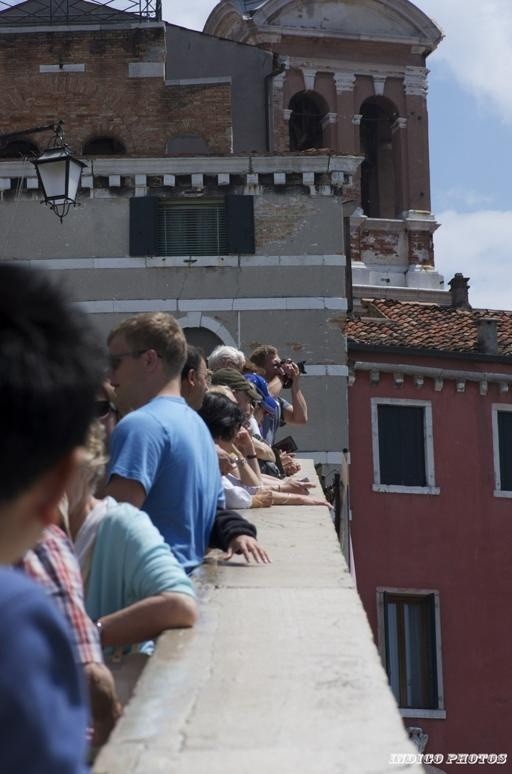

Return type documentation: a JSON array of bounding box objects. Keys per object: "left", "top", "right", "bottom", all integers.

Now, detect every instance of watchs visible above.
[
  {"left": 247, "top": 455, "right": 256, "bottom": 459},
  {"left": 235, "top": 458, "right": 247, "bottom": 468}
]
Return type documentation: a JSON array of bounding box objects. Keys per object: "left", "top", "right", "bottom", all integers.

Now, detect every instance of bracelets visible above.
[
  {"left": 277, "top": 484, "right": 281, "bottom": 494},
  {"left": 283, "top": 491, "right": 291, "bottom": 506}
]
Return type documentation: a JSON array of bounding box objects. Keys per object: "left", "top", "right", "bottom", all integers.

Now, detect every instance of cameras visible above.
[{"left": 272, "top": 359, "right": 307, "bottom": 381}]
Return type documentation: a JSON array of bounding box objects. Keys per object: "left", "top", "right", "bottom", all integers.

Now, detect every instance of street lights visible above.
[{"left": 0, "top": 119, "right": 88, "bottom": 224}]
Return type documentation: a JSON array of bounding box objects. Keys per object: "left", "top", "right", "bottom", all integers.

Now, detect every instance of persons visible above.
[
  {"left": 64, "top": 420, "right": 196, "bottom": 661},
  {"left": 102, "top": 313, "right": 220, "bottom": 576},
  {"left": 16, "top": 446, "right": 122, "bottom": 747},
  {"left": 173, "top": 338, "right": 333, "bottom": 565},
  {"left": 0, "top": 253, "right": 97, "bottom": 769}
]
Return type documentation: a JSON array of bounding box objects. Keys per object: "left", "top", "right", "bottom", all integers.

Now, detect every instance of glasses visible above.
[{"left": 106, "top": 352, "right": 146, "bottom": 369}]
